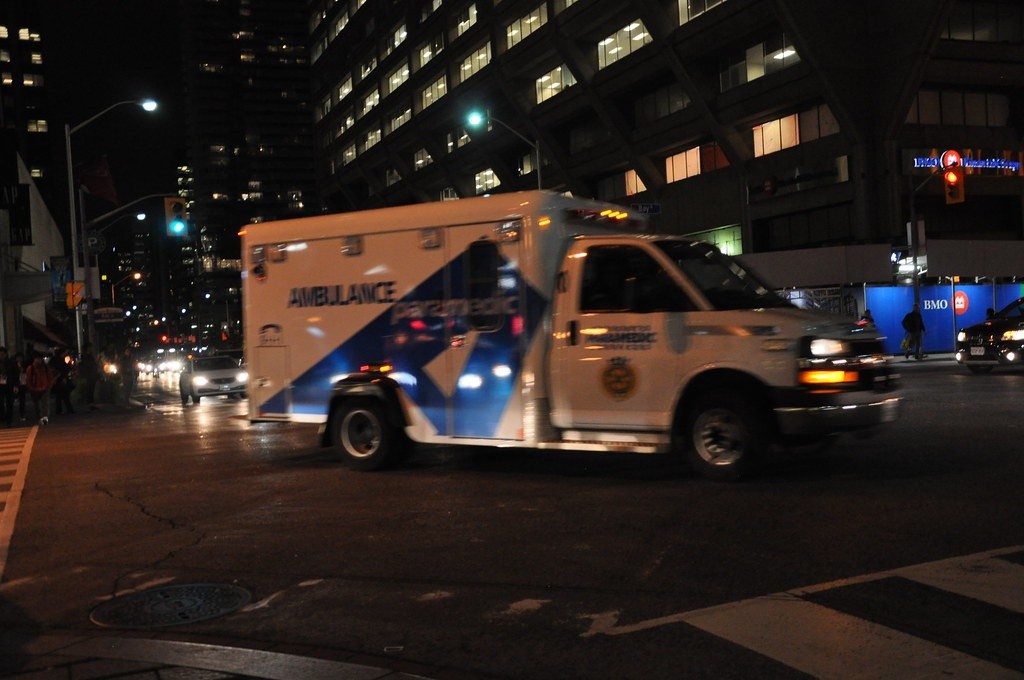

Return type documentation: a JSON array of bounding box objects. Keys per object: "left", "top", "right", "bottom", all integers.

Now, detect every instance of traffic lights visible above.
[{"left": 945, "top": 169, "right": 964, "bottom": 204}]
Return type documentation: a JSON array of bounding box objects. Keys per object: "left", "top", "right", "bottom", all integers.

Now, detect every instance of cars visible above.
[
  {"left": 180, "top": 355, "right": 247, "bottom": 405},
  {"left": 955, "top": 297, "right": 1024, "bottom": 373}
]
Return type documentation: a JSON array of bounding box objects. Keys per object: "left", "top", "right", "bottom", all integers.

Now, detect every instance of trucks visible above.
[{"left": 239, "top": 189, "right": 904, "bottom": 481}]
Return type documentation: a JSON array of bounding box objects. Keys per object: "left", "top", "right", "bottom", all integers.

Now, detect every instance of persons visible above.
[
  {"left": 592, "top": 255, "right": 646, "bottom": 308},
  {"left": 861, "top": 310, "right": 875, "bottom": 327},
  {"left": 0, "top": 343, "right": 76, "bottom": 426},
  {"left": 902, "top": 304, "right": 927, "bottom": 359},
  {"left": 81, "top": 343, "right": 100, "bottom": 412},
  {"left": 121, "top": 347, "right": 138, "bottom": 410}
]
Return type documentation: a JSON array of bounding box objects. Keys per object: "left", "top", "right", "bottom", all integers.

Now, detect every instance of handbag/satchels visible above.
[{"left": 900, "top": 334, "right": 911, "bottom": 351}]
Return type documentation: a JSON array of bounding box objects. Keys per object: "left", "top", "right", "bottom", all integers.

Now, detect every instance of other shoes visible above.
[
  {"left": 905, "top": 353, "right": 908, "bottom": 358},
  {"left": 915, "top": 355, "right": 919, "bottom": 359}
]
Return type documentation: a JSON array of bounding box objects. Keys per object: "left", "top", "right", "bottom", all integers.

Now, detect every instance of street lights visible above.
[
  {"left": 64, "top": 100, "right": 157, "bottom": 358},
  {"left": 165, "top": 198, "right": 187, "bottom": 237},
  {"left": 469, "top": 113, "right": 542, "bottom": 190},
  {"left": 111, "top": 273, "right": 141, "bottom": 305}
]
